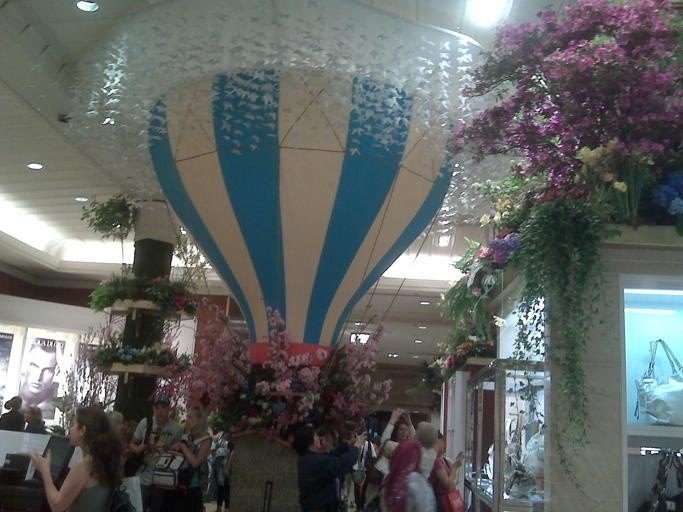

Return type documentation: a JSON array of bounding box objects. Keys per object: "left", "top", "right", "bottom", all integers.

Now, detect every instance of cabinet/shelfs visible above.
[{"left": 461, "top": 358, "right": 545, "bottom": 511}]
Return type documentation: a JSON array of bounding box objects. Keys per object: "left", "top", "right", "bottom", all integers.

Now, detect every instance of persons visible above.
[
  {"left": 17, "top": 337, "right": 77, "bottom": 420},
  {"left": 278, "top": 406, "right": 463, "bottom": 512},
  {"left": 22, "top": 406, "right": 47, "bottom": 434},
  {"left": 0, "top": 396, "right": 24, "bottom": 431},
  {"left": 28, "top": 390, "right": 235, "bottom": 510}
]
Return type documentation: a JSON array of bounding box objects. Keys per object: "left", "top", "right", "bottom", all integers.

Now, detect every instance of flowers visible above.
[
  {"left": 78, "top": 190, "right": 137, "bottom": 242},
  {"left": 86, "top": 276, "right": 198, "bottom": 375},
  {"left": 165, "top": 296, "right": 393, "bottom": 459}
]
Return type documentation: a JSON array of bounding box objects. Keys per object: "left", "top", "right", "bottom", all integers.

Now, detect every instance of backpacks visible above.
[{"left": 152, "top": 433, "right": 212, "bottom": 491}]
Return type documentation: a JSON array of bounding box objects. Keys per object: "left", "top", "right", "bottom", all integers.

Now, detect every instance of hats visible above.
[{"left": 154, "top": 394, "right": 171, "bottom": 405}]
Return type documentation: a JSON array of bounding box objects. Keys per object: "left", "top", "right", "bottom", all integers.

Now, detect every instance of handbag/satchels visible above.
[
  {"left": 124, "top": 449, "right": 144, "bottom": 477},
  {"left": 635, "top": 340, "right": 683, "bottom": 426},
  {"left": 365, "top": 457, "right": 384, "bottom": 486},
  {"left": 440, "top": 490, "right": 466, "bottom": 512},
  {"left": 637, "top": 450, "right": 682, "bottom": 512},
  {"left": 105, "top": 478, "right": 137, "bottom": 512}
]
